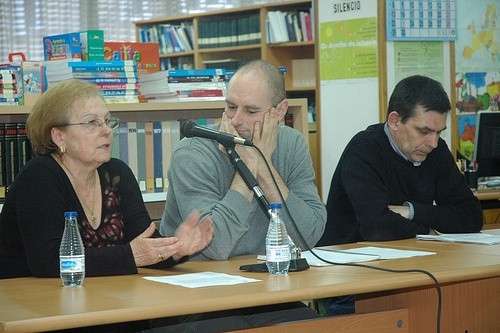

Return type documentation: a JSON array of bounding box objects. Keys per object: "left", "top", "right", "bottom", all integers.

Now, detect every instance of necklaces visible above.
[{"left": 57, "top": 154, "right": 97, "bottom": 225}]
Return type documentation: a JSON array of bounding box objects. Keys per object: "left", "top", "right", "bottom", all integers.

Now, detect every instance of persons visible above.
[
  {"left": 0, "top": 81, "right": 214, "bottom": 279},
  {"left": 159, "top": 59, "right": 329, "bottom": 333},
  {"left": 314, "top": 76, "right": 484, "bottom": 315}
]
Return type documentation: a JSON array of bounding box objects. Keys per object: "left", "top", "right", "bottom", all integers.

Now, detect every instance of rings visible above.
[{"left": 160, "top": 253, "right": 165, "bottom": 258}]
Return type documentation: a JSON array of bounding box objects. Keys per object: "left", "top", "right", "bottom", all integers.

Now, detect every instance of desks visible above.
[{"left": 0, "top": 227, "right": 500, "bottom": 333}]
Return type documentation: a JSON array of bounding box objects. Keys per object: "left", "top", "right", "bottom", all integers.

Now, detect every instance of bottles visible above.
[
  {"left": 59, "top": 211, "right": 85, "bottom": 287},
  {"left": 265, "top": 203, "right": 291, "bottom": 275}
]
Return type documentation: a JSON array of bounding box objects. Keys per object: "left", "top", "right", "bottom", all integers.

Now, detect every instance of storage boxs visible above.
[{"left": 42, "top": 30, "right": 161, "bottom": 72}]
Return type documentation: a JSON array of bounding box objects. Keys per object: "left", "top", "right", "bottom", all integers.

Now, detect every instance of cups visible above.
[{"left": 465, "top": 170, "right": 478, "bottom": 193}]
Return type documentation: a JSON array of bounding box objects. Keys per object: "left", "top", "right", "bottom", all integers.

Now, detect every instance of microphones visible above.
[{"left": 179, "top": 121, "right": 253, "bottom": 146}]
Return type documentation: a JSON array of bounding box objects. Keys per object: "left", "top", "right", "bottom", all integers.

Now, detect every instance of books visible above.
[{"left": 0, "top": 8, "right": 315, "bottom": 206}]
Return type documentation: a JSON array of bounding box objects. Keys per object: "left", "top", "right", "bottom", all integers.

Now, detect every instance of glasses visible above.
[{"left": 61, "top": 117, "right": 121, "bottom": 131}]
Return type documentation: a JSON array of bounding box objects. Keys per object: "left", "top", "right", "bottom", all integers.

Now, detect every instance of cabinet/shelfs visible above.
[{"left": 135, "top": 0, "right": 321, "bottom": 199}]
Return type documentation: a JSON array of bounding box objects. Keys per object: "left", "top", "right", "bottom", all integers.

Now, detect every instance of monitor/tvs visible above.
[{"left": 474, "top": 110, "right": 500, "bottom": 180}]
